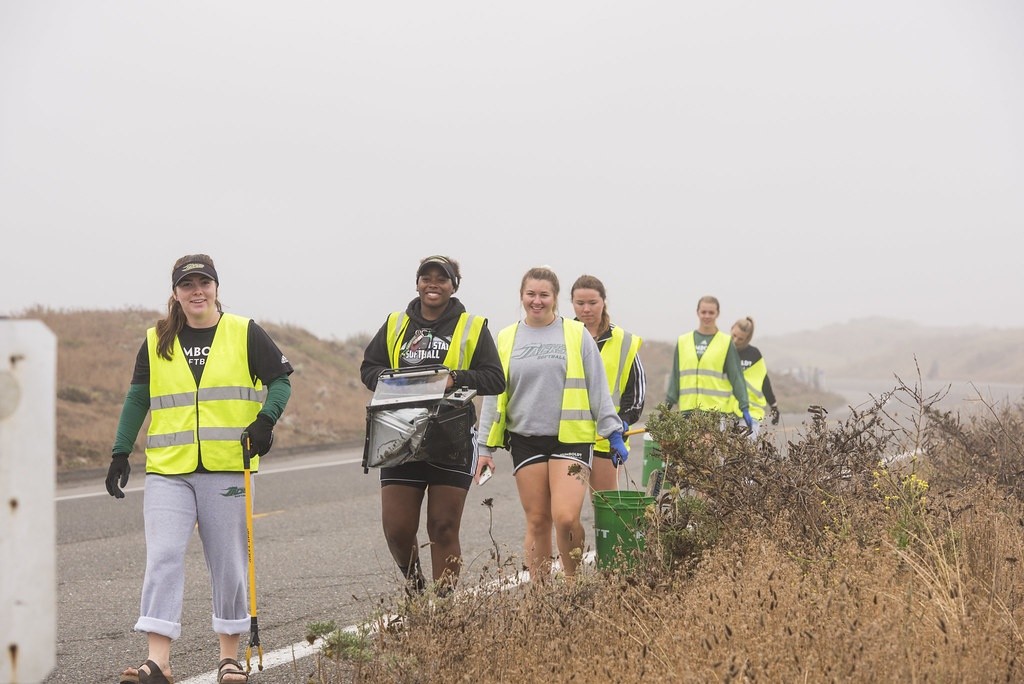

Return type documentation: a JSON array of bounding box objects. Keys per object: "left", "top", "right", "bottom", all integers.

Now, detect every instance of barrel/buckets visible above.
[
  {"left": 642, "top": 432, "right": 673, "bottom": 489},
  {"left": 592, "top": 457, "right": 655, "bottom": 571}
]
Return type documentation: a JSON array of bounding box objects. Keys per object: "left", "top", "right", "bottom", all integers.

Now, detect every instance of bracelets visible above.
[{"left": 449, "top": 372, "right": 457, "bottom": 387}]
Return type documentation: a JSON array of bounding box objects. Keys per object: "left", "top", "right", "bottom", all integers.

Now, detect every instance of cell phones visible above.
[{"left": 479, "top": 464, "right": 492, "bottom": 486}]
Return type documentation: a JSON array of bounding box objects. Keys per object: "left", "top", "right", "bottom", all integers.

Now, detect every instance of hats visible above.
[
  {"left": 415, "top": 256, "right": 457, "bottom": 289},
  {"left": 171, "top": 262, "right": 218, "bottom": 288}
]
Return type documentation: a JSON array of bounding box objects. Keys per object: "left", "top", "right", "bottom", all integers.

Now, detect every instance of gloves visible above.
[
  {"left": 742, "top": 408, "right": 753, "bottom": 427},
  {"left": 622, "top": 420, "right": 631, "bottom": 442},
  {"left": 241, "top": 413, "right": 275, "bottom": 459},
  {"left": 609, "top": 431, "right": 628, "bottom": 468},
  {"left": 105, "top": 452, "right": 131, "bottom": 498},
  {"left": 772, "top": 408, "right": 780, "bottom": 426}
]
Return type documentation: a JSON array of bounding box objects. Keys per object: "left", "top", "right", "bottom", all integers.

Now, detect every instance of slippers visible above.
[
  {"left": 217, "top": 658, "right": 248, "bottom": 684},
  {"left": 120, "top": 659, "right": 174, "bottom": 684}
]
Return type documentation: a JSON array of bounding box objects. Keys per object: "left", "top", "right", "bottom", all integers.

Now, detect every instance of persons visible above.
[
  {"left": 658, "top": 295, "right": 753, "bottom": 500},
  {"left": 570, "top": 274, "right": 646, "bottom": 502},
  {"left": 724, "top": 317, "right": 780, "bottom": 486},
  {"left": 104, "top": 254, "right": 293, "bottom": 684},
  {"left": 473, "top": 267, "right": 628, "bottom": 581},
  {"left": 359, "top": 256, "right": 506, "bottom": 598}
]
